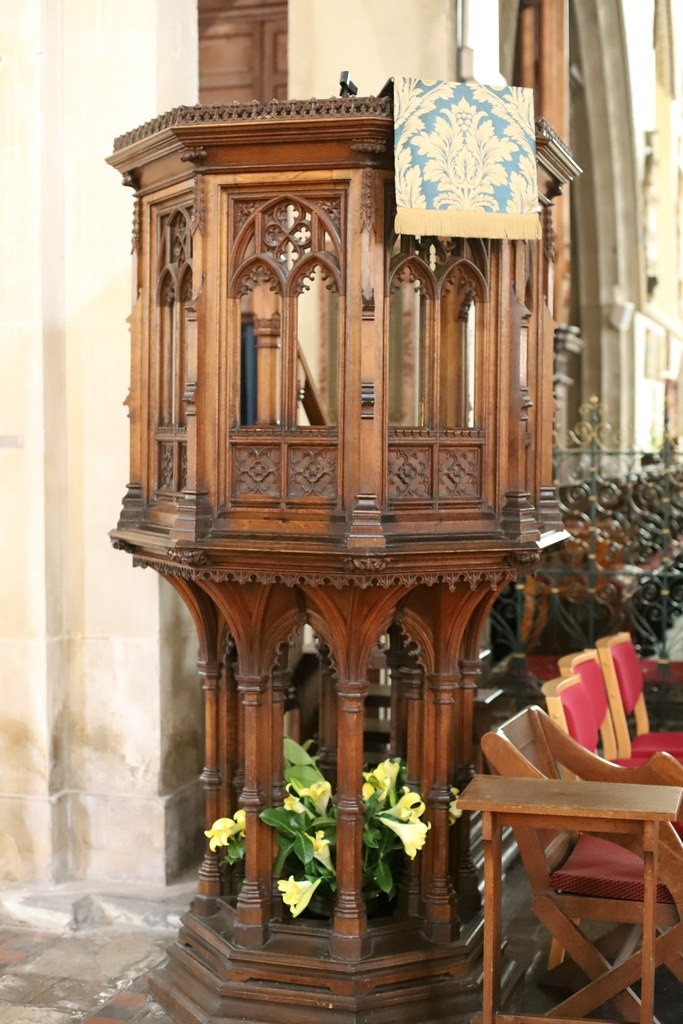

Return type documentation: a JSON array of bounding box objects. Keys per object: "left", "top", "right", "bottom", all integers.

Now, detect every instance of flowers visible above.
[{"left": 204, "top": 734, "right": 464, "bottom": 918}]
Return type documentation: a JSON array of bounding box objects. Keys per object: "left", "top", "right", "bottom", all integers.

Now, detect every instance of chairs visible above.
[{"left": 480, "top": 631, "right": 683, "bottom": 1001}]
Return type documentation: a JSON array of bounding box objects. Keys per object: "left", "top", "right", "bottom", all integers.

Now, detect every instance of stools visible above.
[{"left": 457, "top": 773, "right": 683, "bottom": 1024}]
